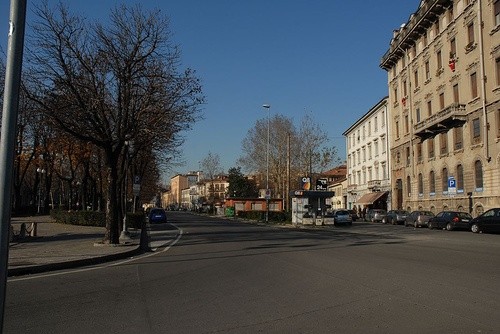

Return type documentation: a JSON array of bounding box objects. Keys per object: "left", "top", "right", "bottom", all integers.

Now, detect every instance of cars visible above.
[
  {"left": 468, "top": 208, "right": 500, "bottom": 235},
  {"left": 381, "top": 210, "right": 410, "bottom": 225},
  {"left": 326, "top": 208, "right": 357, "bottom": 227},
  {"left": 428, "top": 211, "right": 474, "bottom": 231},
  {"left": 404, "top": 211, "right": 435, "bottom": 228},
  {"left": 148, "top": 207, "right": 167, "bottom": 225},
  {"left": 365, "top": 209, "right": 387, "bottom": 223}
]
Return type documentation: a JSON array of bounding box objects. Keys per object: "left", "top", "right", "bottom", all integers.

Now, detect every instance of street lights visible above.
[
  {"left": 261, "top": 104, "right": 271, "bottom": 223},
  {"left": 36, "top": 167, "right": 45, "bottom": 214}
]
